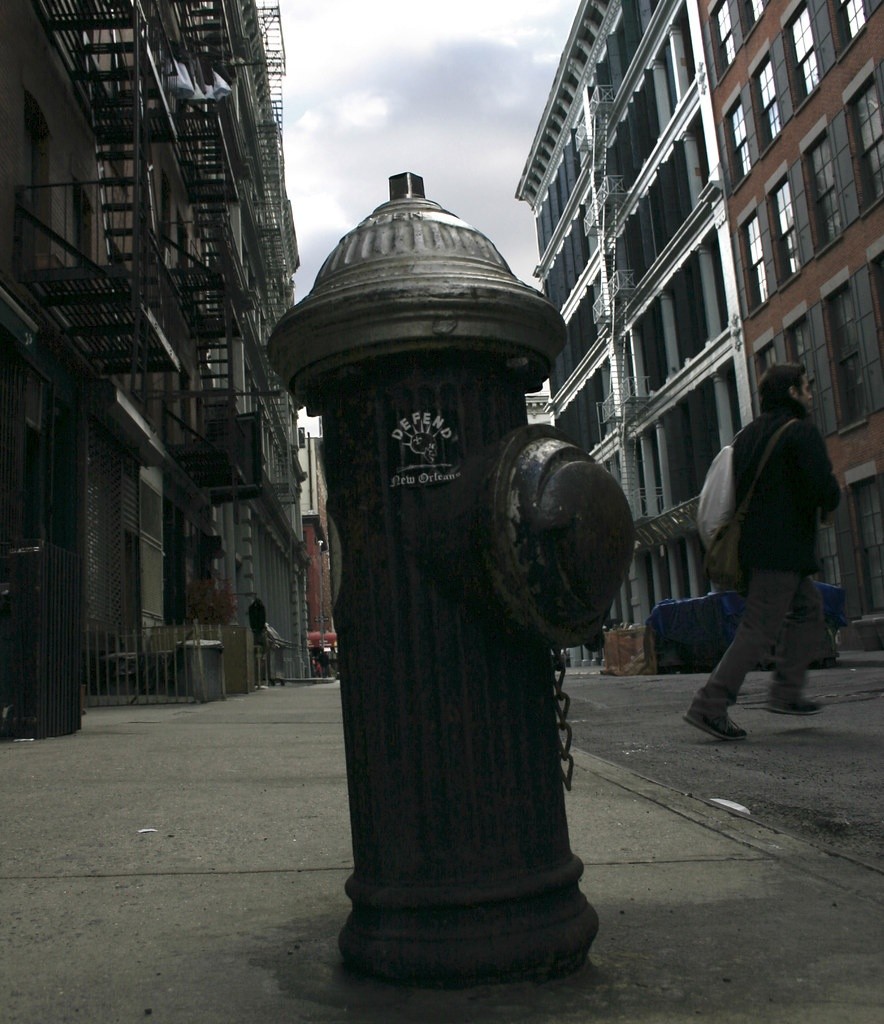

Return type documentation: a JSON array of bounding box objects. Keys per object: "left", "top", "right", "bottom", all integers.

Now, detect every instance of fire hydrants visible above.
[{"left": 266, "top": 171, "right": 637, "bottom": 983}]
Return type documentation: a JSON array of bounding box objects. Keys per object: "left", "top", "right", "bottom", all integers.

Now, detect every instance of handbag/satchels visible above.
[
  {"left": 698, "top": 446, "right": 736, "bottom": 549},
  {"left": 700, "top": 523, "right": 743, "bottom": 590}
]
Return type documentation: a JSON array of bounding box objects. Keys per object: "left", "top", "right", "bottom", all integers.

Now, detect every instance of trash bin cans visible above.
[{"left": 177, "top": 638, "right": 225, "bottom": 703}]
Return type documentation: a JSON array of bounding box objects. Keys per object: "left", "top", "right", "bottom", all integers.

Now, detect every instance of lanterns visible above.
[{"left": 308, "top": 631, "right": 336, "bottom": 647}]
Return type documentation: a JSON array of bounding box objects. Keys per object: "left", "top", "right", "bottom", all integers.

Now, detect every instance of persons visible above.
[
  {"left": 319, "top": 652, "right": 331, "bottom": 678},
  {"left": 682, "top": 363, "right": 842, "bottom": 741}
]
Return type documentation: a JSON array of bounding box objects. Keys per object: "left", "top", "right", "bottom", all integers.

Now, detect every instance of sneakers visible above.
[
  {"left": 683, "top": 710, "right": 747, "bottom": 739},
  {"left": 766, "top": 700, "right": 826, "bottom": 714}
]
